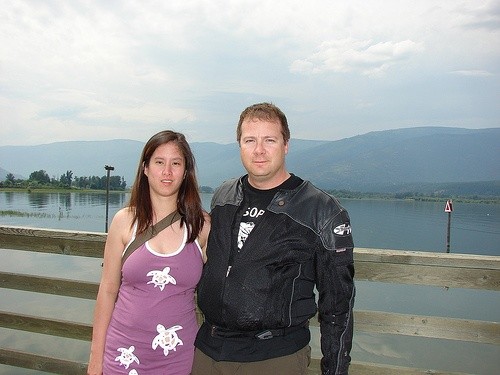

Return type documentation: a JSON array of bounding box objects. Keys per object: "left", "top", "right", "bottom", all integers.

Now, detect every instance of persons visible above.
[
  {"left": 191, "top": 103, "right": 355, "bottom": 375},
  {"left": 87, "top": 129, "right": 212, "bottom": 375}
]
